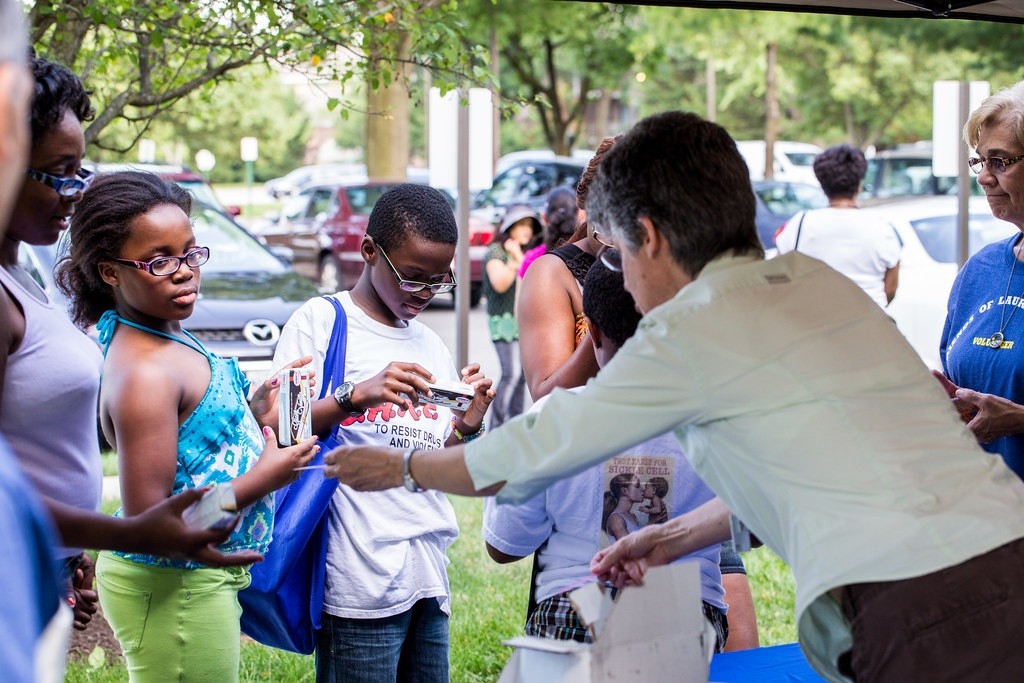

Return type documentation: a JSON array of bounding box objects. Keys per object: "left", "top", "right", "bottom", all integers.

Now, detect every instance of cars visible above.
[
  {"left": 733, "top": 135, "right": 1023, "bottom": 376},
  {"left": 12, "top": 147, "right": 602, "bottom": 404}
]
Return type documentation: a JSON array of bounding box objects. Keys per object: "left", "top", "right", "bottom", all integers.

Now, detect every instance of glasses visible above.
[
  {"left": 364, "top": 234, "right": 458, "bottom": 294},
  {"left": 969, "top": 153, "right": 1024, "bottom": 174},
  {"left": 108, "top": 246, "right": 210, "bottom": 277},
  {"left": 593, "top": 226, "right": 618, "bottom": 249},
  {"left": 596, "top": 245, "right": 623, "bottom": 273},
  {"left": 27, "top": 167, "right": 95, "bottom": 198}
]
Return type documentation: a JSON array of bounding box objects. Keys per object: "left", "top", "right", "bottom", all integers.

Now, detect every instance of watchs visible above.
[
  {"left": 334, "top": 381, "right": 364, "bottom": 418},
  {"left": 402, "top": 447, "right": 427, "bottom": 492}
]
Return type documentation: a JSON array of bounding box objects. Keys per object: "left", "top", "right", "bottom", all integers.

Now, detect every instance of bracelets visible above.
[{"left": 451, "top": 415, "right": 485, "bottom": 443}]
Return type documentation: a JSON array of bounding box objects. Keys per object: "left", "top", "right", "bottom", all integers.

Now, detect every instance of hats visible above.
[{"left": 500, "top": 207, "right": 543, "bottom": 236}]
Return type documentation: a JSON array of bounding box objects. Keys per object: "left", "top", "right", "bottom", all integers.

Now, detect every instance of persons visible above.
[
  {"left": 482, "top": 209, "right": 544, "bottom": 430},
  {"left": 775, "top": 144, "right": 904, "bottom": 310},
  {"left": 0, "top": 0, "right": 266, "bottom": 683},
  {"left": 275, "top": 182, "right": 497, "bottom": 683},
  {"left": 929, "top": 80, "right": 1024, "bottom": 480},
  {"left": 481, "top": 247, "right": 730, "bottom": 653},
  {"left": 324, "top": 111, "right": 1024, "bottom": 683},
  {"left": 55, "top": 172, "right": 321, "bottom": 683},
  {"left": 516, "top": 185, "right": 578, "bottom": 283},
  {"left": 602, "top": 472, "right": 669, "bottom": 544},
  {"left": 517, "top": 135, "right": 760, "bottom": 652}
]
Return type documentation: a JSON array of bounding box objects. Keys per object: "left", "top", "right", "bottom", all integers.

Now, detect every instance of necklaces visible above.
[{"left": 990, "top": 240, "right": 1024, "bottom": 348}]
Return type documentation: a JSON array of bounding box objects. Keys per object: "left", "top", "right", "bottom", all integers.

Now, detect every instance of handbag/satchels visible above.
[{"left": 238, "top": 437, "right": 344, "bottom": 656}]
